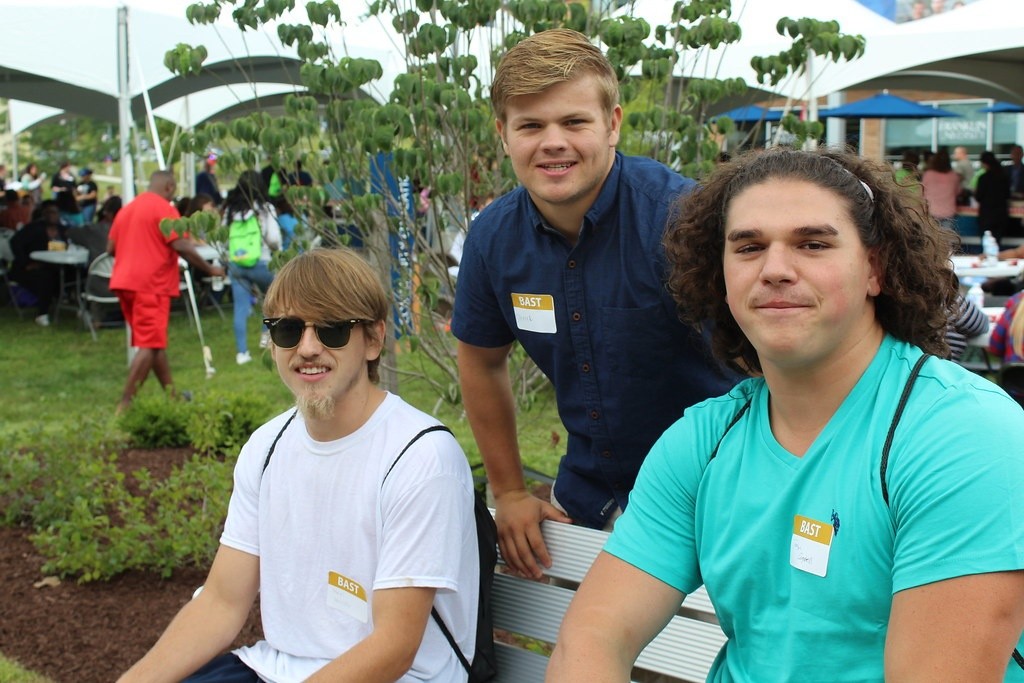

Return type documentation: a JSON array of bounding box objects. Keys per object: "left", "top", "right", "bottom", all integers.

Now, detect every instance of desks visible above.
[
  {"left": 948, "top": 255, "right": 1024, "bottom": 380},
  {"left": 30, "top": 248, "right": 89, "bottom": 331}
]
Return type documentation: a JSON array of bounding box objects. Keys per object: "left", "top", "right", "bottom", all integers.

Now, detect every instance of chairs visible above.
[
  {"left": 0, "top": 229, "right": 25, "bottom": 319},
  {"left": 79, "top": 246, "right": 230, "bottom": 341}
]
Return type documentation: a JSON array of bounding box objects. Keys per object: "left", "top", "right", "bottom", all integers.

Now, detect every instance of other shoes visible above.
[
  {"left": 260, "top": 334, "right": 271, "bottom": 348},
  {"left": 235, "top": 350, "right": 253, "bottom": 364},
  {"left": 172, "top": 390, "right": 193, "bottom": 404},
  {"left": 35, "top": 313, "right": 49, "bottom": 327}
]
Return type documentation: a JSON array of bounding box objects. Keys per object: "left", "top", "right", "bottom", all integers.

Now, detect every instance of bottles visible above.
[
  {"left": 981, "top": 230, "right": 999, "bottom": 269},
  {"left": 211, "top": 257, "right": 224, "bottom": 292},
  {"left": 966, "top": 282, "right": 986, "bottom": 314}
]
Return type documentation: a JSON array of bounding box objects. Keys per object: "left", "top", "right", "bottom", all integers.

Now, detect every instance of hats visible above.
[{"left": 78, "top": 167, "right": 93, "bottom": 176}]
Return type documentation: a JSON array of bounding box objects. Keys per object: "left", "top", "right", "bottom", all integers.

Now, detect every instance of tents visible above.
[
  {"left": 1, "top": 0, "right": 533, "bottom": 213},
  {"left": 590, "top": 0, "right": 1024, "bottom": 146}
]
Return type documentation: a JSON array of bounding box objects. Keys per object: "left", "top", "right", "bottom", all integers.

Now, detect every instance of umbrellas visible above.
[{"left": 711, "top": 88, "right": 1024, "bottom": 163}]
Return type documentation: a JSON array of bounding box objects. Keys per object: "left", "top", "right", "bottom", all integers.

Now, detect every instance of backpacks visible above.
[
  {"left": 227, "top": 200, "right": 264, "bottom": 269},
  {"left": 261, "top": 406, "right": 498, "bottom": 682}
]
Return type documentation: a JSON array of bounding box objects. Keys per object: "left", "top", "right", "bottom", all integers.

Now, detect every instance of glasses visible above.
[{"left": 263, "top": 317, "right": 374, "bottom": 350}]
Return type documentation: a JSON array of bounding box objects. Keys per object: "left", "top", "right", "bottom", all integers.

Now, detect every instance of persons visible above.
[
  {"left": 0, "top": 156, "right": 506, "bottom": 326},
  {"left": 545, "top": 148, "right": 1024, "bottom": 683},
  {"left": 450, "top": 32, "right": 763, "bottom": 580},
  {"left": 892, "top": 145, "right": 1024, "bottom": 409},
  {"left": 106, "top": 166, "right": 226, "bottom": 419},
  {"left": 223, "top": 171, "right": 284, "bottom": 365},
  {"left": 113, "top": 249, "right": 484, "bottom": 683}
]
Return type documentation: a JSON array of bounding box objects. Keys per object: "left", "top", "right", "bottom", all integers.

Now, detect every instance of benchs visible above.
[{"left": 488, "top": 507, "right": 728, "bottom": 683}]
